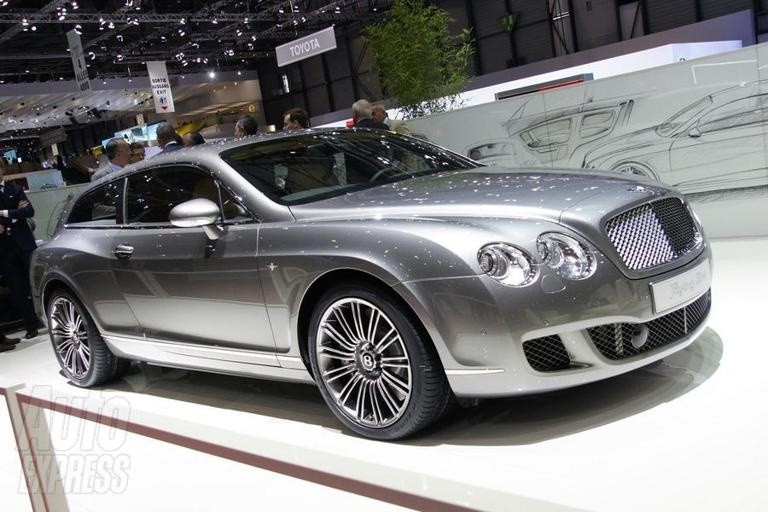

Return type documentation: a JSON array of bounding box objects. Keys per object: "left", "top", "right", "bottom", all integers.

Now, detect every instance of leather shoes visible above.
[
  {"left": 0, "top": 337, "right": 21, "bottom": 352},
  {"left": 26, "top": 328, "right": 37, "bottom": 339}
]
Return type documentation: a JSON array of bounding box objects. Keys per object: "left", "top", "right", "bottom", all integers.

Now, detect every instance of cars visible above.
[{"left": 25, "top": 126, "right": 718, "bottom": 442}]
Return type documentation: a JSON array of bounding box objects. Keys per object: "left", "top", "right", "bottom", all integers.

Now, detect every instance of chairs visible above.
[
  {"left": 287, "top": 161, "right": 342, "bottom": 195},
  {"left": 193, "top": 174, "right": 279, "bottom": 219}
]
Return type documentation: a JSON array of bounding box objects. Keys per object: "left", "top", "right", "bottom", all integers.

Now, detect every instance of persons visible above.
[
  {"left": 278, "top": 108, "right": 339, "bottom": 185},
  {"left": 330, "top": 99, "right": 391, "bottom": 186},
  {"left": 0, "top": 330, "right": 21, "bottom": 352},
  {"left": 233, "top": 116, "right": 276, "bottom": 184},
  {"left": 0, "top": 157, "right": 39, "bottom": 339},
  {"left": 54, "top": 122, "right": 207, "bottom": 185}
]
